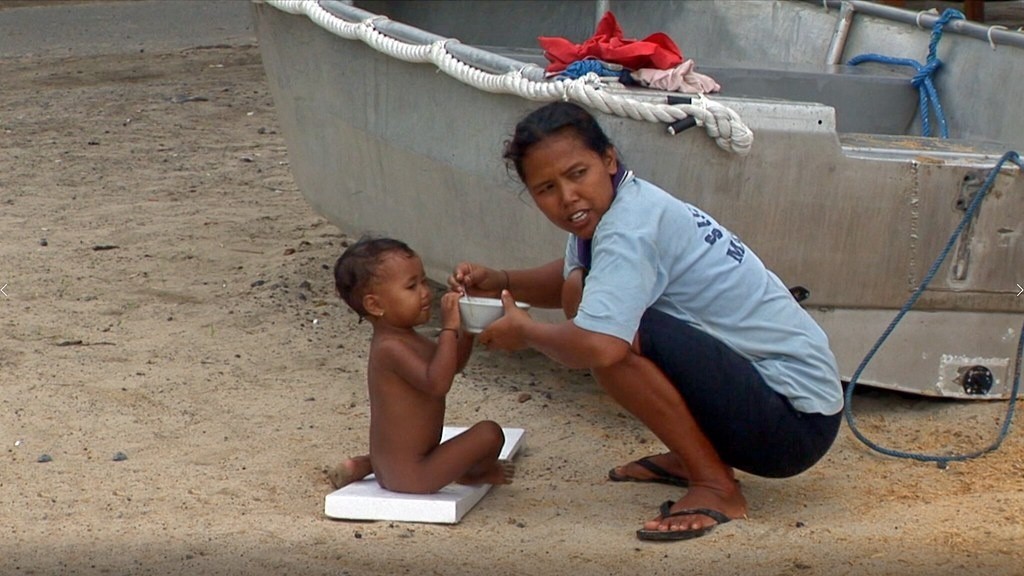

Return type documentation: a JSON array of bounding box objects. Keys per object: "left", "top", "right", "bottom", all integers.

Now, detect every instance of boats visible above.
[{"left": 248, "top": 0, "right": 1024, "bottom": 402}]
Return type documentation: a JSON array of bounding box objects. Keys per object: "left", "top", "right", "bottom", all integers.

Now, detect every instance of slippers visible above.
[
  {"left": 608, "top": 454, "right": 691, "bottom": 486},
  {"left": 636, "top": 499, "right": 729, "bottom": 540}
]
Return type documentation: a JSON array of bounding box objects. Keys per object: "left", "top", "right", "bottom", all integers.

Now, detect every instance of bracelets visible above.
[
  {"left": 436, "top": 328, "right": 459, "bottom": 339},
  {"left": 502, "top": 269, "right": 510, "bottom": 289}
]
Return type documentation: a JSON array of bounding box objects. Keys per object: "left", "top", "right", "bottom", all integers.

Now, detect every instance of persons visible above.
[
  {"left": 447, "top": 101, "right": 844, "bottom": 541},
  {"left": 328, "top": 237, "right": 516, "bottom": 494}
]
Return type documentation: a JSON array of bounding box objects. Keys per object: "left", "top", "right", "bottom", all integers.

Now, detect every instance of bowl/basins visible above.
[{"left": 458, "top": 296, "right": 531, "bottom": 335}]
